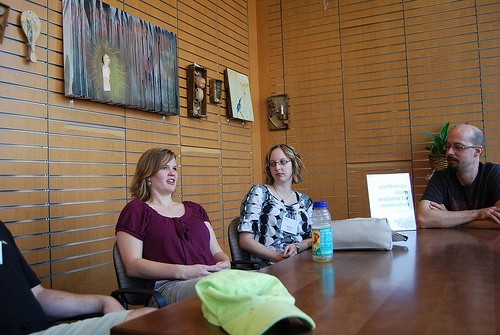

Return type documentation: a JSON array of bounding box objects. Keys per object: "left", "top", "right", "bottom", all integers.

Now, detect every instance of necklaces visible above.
[{"left": 273, "top": 184, "right": 291, "bottom": 203}]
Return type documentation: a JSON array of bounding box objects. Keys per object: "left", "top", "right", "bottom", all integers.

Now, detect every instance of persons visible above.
[
  {"left": 0, "top": 219, "right": 161, "bottom": 335},
  {"left": 114, "top": 149, "right": 230, "bottom": 306},
  {"left": 195, "top": 77, "right": 205, "bottom": 115},
  {"left": 417, "top": 125, "right": 500, "bottom": 229},
  {"left": 238, "top": 144, "right": 315, "bottom": 270}
]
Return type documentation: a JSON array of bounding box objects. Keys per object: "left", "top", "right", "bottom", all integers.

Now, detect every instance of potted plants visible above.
[{"left": 423, "top": 123, "right": 449, "bottom": 172}]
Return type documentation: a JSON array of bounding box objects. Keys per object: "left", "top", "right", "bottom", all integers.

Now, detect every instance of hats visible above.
[{"left": 195, "top": 269, "right": 316, "bottom": 335}]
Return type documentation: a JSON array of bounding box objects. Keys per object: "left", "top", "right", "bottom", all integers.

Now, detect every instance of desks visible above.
[{"left": 110, "top": 228, "right": 500, "bottom": 335}]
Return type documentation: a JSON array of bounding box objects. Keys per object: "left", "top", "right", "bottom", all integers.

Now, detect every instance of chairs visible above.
[
  {"left": 110, "top": 240, "right": 167, "bottom": 309},
  {"left": 228, "top": 217, "right": 268, "bottom": 271}
]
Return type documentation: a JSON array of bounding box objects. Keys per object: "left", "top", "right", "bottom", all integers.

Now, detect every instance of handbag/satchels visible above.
[{"left": 330, "top": 218, "right": 408, "bottom": 251}]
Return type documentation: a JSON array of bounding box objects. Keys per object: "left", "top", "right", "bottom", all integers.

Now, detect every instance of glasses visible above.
[
  {"left": 269, "top": 159, "right": 291, "bottom": 167},
  {"left": 444, "top": 142, "right": 478, "bottom": 152}
]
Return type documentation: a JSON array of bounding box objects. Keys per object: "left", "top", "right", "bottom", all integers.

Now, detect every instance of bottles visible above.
[{"left": 311, "top": 201, "right": 334, "bottom": 262}]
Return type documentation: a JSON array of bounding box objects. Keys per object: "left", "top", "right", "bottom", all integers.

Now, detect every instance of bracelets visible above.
[{"left": 295, "top": 244, "right": 299, "bottom": 254}]
